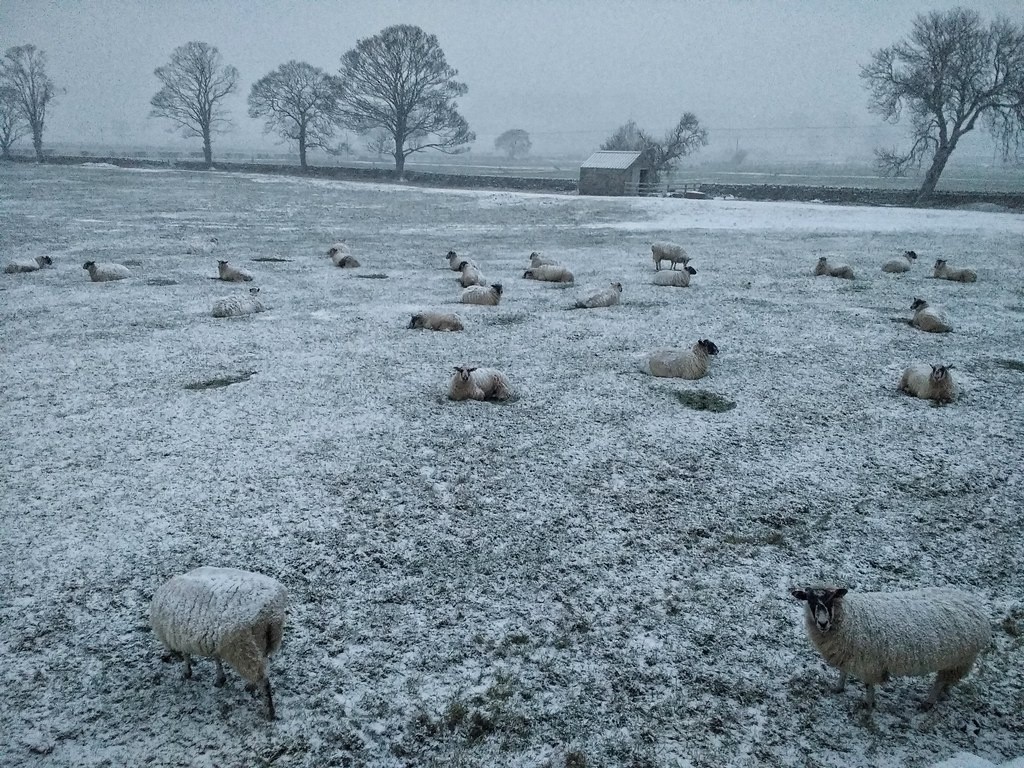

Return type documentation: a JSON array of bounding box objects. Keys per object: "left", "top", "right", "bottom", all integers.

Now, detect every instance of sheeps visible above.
[
  {"left": 908, "top": 295, "right": 956, "bottom": 334},
  {"left": 651, "top": 240, "right": 693, "bottom": 271},
  {"left": 897, "top": 361, "right": 960, "bottom": 404},
  {"left": 791, "top": 584, "right": 992, "bottom": 713},
  {"left": 575, "top": 281, "right": 623, "bottom": 308},
  {"left": 446, "top": 250, "right": 504, "bottom": 306},
  {"left": 522, "top": 251, "right": 576, "bottom": 283},
  {"left": 140, "top": 566, "right": 287, "bottom": 722},
  {"left": 445, "top": 366, "right": 515, "bottom": 402},
  {"left": 406, "top": 310, "right": 465, "bottom": 333},
  {"left": 653, "top": 265, "right": 697, "bottom": 287},
  {"left": 813, "top": 256, "right": 855, "bottom": 280},
  {"left": 636, "top": 335, "right": 720, "bottom": 380},
  {"left": 4, "top": 255, "right": 53, "bottom": 274},
  {"left": 325, "top": 239, "right": 361, "bottom": 269},
  {"left": 211, "top": 287, "right": 268, "bottom": 318},
  {"left": 210, "top": 260, "right": 254, "bottom": 282},
  {"left": 924, "top": 258, "right": 977, "bottom": 283},
  {"left": 83, "top": 260, "right": 133, "bottom": 282},
  {"left": 880, "top": 249, "right": 918, "bottom": 273}
]
